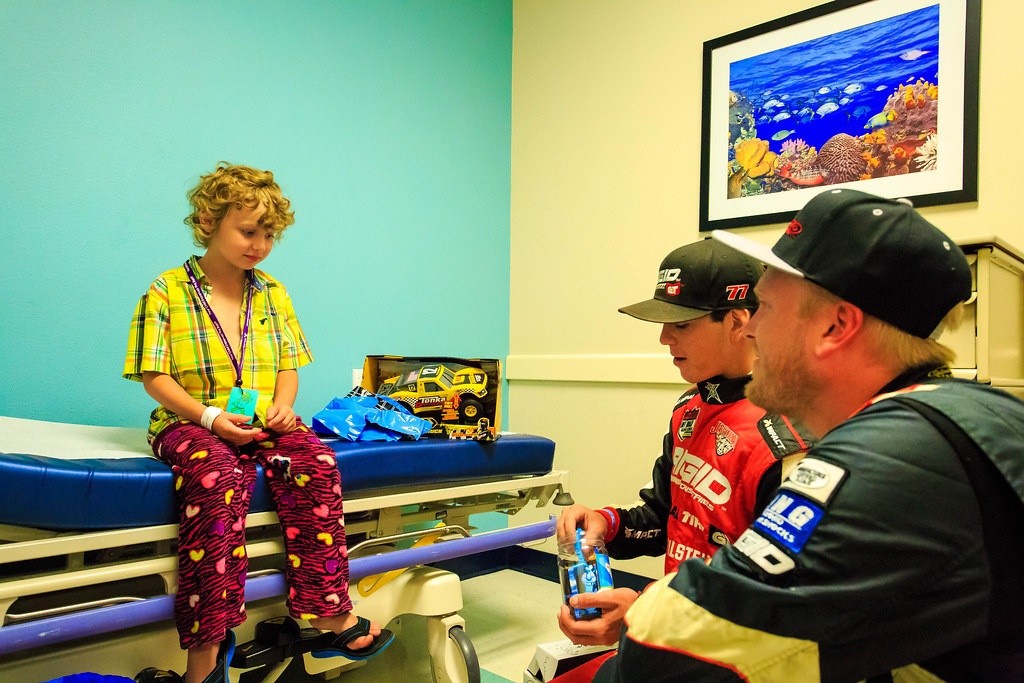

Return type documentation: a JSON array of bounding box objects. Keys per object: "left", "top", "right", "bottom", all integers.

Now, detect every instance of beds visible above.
[{"left": 0, "top": 416, "right": 574, "bottom": 683}]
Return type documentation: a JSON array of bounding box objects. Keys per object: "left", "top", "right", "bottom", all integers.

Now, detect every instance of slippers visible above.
[
  {"left": 311, "top": 617, "right": 395, "bottom": 661},
  {"left": 181, "top": 629, "right": 236, "bottom": 682}
]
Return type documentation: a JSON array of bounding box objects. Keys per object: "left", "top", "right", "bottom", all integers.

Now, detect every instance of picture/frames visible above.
[{"left": 698, "top": 0, "right": 981, "bottom": 232}]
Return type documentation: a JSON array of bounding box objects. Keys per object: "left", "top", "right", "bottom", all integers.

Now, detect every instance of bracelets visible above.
[{"left": 201, "top": 406, "right": 221, "bottom": 432}]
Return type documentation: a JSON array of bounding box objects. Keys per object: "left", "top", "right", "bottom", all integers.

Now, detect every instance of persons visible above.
[
  {"left": 547, "top": 237, "right": 819, "bottom": 683},
  {"left": 124, "top": 166, "right": 394, "bottom": 683},
  {"left": 617, "top": 189, "right": 1024, "bottom": 683}
]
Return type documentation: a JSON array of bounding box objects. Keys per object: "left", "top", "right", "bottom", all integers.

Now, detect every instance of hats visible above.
[
  {"left": 619, "top": 235, "right": 764, "bottom": 324},
  {"left": 710, "top": 188, "right": 973, "bottom": 341}
]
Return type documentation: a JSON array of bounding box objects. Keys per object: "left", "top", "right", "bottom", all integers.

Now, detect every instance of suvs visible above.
[{"left": 376, "top": 365, "right": 488, "bottom": 423}]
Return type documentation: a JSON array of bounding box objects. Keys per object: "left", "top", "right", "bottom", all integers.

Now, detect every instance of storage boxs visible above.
[{"left": 361, "top": 353, "right": 503, "bottom": 442}]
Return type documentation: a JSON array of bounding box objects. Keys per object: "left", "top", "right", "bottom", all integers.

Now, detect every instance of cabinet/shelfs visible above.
[{"left": 945, "top": 239, "right": 1024, "bottom": 387}]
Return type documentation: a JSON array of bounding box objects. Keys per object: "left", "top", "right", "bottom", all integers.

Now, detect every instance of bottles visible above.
[{"left": 557, "top": 531, "right": 617, "bottom": 620}]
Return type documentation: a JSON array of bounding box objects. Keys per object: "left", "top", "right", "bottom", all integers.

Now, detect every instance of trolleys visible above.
[{"left": 0, "top": 470, "right": 575, "bottom": 683}]
